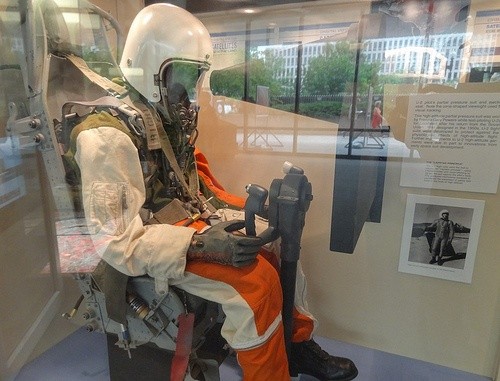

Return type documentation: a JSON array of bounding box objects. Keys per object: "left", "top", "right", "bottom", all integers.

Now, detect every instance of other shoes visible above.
[
  {"left": 438, "top": 256, "right": 442, "bottom": 266},
  {"left": 429, "top": 256, "right": 436, "bottom": 264}
]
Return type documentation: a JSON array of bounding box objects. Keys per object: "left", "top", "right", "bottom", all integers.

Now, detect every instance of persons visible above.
[
  {"left": 426, "top": 209, "right": 458, "bottom": 265},
  {"left": 341, "top": 98, "right": 363, "bottom": 138},
  {"left": 371, "top": 99, "right": 386, "bottom": 131},
  {"left": 66, "top": 3, "right": 359, "bottom": 381}
]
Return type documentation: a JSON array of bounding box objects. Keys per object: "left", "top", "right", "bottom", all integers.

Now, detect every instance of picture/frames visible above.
[{"left": 397, "top": 194, "right": 486, "bottom": 284}]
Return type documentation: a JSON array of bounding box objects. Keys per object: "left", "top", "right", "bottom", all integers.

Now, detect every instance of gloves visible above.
[{"left": 192, "top": 220, "right": 261, "bottom": 267}]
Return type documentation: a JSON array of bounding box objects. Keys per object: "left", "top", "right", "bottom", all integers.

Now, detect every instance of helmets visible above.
[
  {"left": 439, "top": 210, "right": 449, "bottom": 218},
  {"left": 120, "top": 4, "right": 213, "bottom": 103}
]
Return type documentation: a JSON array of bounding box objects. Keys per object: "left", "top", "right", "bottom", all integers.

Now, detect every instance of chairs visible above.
[{"left": 24, "top": 0, "right": 235, "bottom": 381}]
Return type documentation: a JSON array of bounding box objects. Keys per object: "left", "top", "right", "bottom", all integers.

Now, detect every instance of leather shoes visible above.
[{"left": 291, "top": 338, "right": 358, "bottom": 381}]
